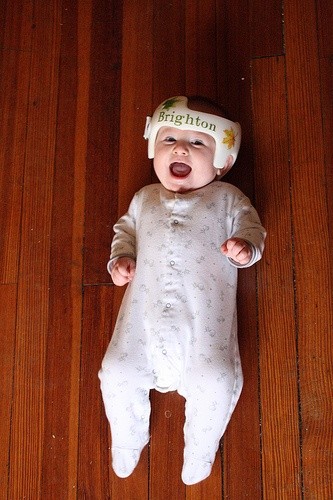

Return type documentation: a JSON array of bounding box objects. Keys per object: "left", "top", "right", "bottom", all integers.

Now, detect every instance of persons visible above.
[{"left": 96, "top": 96, "right": 268, "bottom": 484}]
